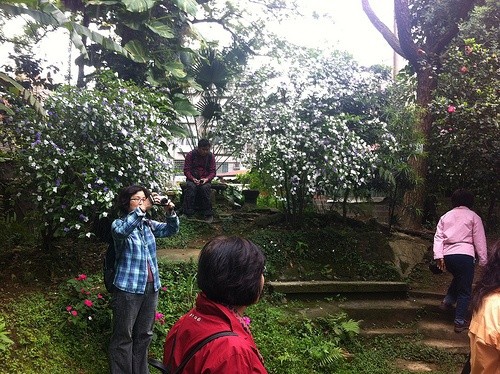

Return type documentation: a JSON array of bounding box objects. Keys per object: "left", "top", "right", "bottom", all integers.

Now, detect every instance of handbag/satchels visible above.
[{"left": 102, "top": 216, "right": 126, "bottom": 293}]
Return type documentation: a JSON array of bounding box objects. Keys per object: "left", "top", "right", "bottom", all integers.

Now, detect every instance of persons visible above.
[
  {"left": 466, "top": 240, "right": 500, "bottom": 374},
  {"left": 101, "top": 185, "right": 180, "bottom": 374},
  {"left": 181, "top": 138, "right": 216, "bottom": 220},
  {"left": 161, "top": 234, "right": 271, "bottom": 374},
  {"left": 433, "top": 187, "right": 488, "bottom": 333}
]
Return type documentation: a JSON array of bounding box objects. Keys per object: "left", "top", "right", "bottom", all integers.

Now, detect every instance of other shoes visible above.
[
  {"left": 440, "top": 295, "right": 454, "bottom": 311},
  {"left": 181, "top": 214, "right": 188, "bottom": 220},
  {"left": 205, "top": 215, "right": 213, "bottom": 223},
  {"left": 454, "top": 319, "right": 470, "bottom": 331}
]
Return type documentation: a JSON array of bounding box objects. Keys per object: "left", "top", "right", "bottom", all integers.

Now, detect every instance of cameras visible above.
[{"left": 154, "top": 195, "right": 168, "bottom": 206}]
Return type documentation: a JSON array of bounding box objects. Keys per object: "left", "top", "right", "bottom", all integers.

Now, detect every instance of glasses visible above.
[
  {"left": 262, "top": 266, "right": 268, "bottom": 276},
  {"left": 130, "top": 197, "right": 146, "bottom": 204}
]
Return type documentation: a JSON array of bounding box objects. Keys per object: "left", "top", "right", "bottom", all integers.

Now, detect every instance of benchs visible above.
[{"left": 178, "top": 180, "right": 227, "bottom": 206}]
